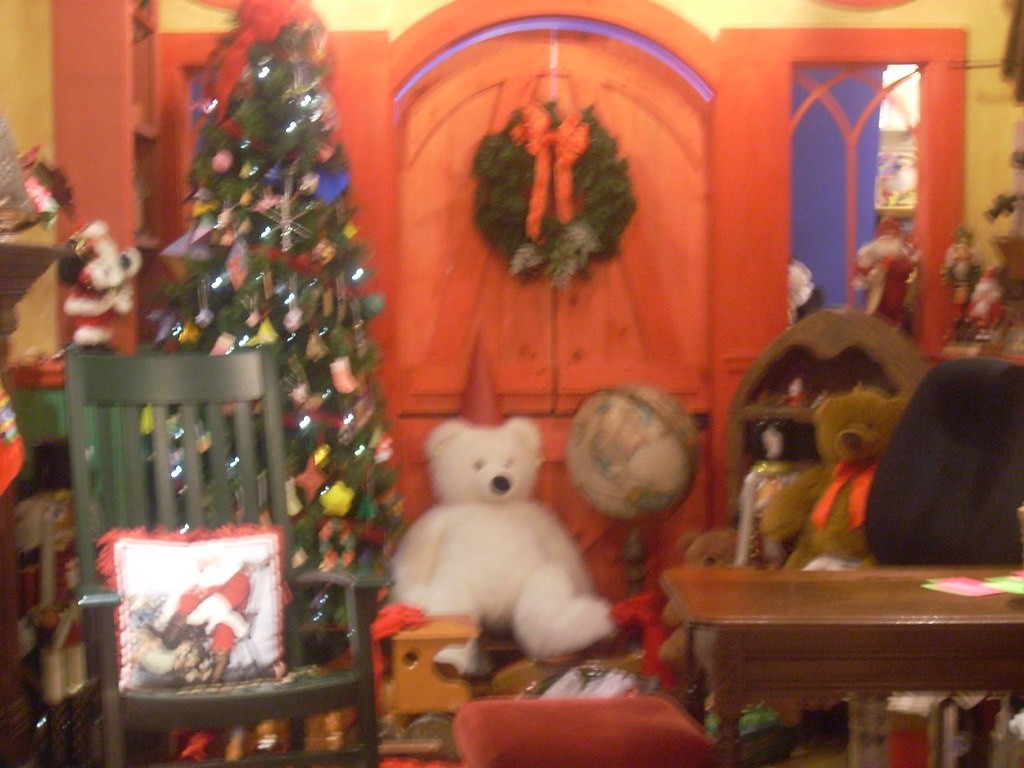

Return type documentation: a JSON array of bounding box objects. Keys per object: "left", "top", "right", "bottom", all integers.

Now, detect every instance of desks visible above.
[{"left": 659, "top": 562, "right": 1024, "bottom": 767}]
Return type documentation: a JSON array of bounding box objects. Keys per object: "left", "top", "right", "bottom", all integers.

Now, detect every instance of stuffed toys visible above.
[
  {"left": 388, "top": 321, "right": 613, "bottom": 676},
  {"left": 660, "top": 526, "right": 736, "bottom": 673},
  {"left": 64, "top": 219, "right": 134, "bottom": 354},
  {"left": 760, "top": 392, "right": 910, "bottom": 572}
]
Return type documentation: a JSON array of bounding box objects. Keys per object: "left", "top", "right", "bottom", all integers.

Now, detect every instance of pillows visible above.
[
  {"left": 96, "top": 522, "right": 288, "bottom": 697},
  {"left": 451, "top": 690, "right": 717, "bottom": 768}
]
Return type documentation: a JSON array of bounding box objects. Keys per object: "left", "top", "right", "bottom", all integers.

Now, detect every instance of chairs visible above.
[
  {"left": 64, "top": 345, "right": 397, "bottom": 768},
  {"left": 864, "top": 359, "right": 1024, "bottom": 562}
]
